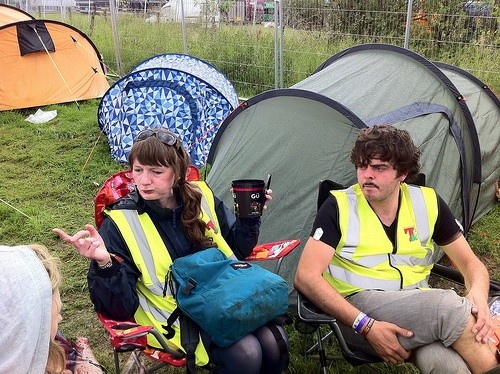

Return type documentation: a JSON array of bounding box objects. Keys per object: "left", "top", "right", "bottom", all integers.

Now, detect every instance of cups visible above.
[{"left": 232, "top": 179, "right": 265, "bottom": 218}]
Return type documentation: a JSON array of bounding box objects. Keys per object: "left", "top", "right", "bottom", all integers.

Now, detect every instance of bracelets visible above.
[
  {"left": 97, "top": 257, "right": 113, "bottom": 269},
  {"left": 351, "top": 311, "right": 376, "bottom": 338}
]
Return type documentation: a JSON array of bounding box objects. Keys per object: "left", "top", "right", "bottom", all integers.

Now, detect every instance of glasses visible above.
[{"left": 131, "top": 130, "right": 182, "bottom": 154}]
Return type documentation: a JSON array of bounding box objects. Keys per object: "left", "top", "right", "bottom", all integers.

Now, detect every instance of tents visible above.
[
  {"left": 0, "top": 3, "right": 111, "bottom": 111},
  {"left": 98, "top": 53, "right": 240, "bottom": 168},
  {"left": 200, "top": 41, "right": 499, "bottom": 319}
]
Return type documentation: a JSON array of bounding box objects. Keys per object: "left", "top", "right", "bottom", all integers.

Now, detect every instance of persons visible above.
[
  {"left": 52, "top": 127, "right": 292, "bottom": 373},
  {"left": 293, "top": 124, "right": 500, "bottom": 374},
  {"left": 0, "top": 241, "right": 107, "bottom": 374}
]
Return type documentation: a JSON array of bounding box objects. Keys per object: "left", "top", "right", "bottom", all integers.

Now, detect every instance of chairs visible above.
[
  {"left": 296, "top": 179, "right": 386, "bottom": 374},
  {"left": 94, "top": 165, "right": 301, "bottom": 374}
]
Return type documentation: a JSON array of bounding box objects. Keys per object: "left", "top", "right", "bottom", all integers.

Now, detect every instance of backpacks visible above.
[{"left": 166, "top": 248, "right": 289, "bottom": 354}]
